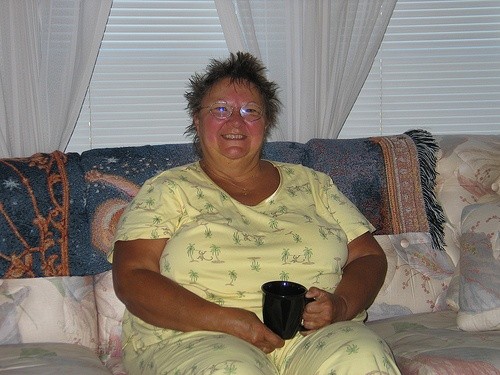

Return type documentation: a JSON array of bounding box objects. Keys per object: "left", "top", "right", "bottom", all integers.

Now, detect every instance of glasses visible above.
[{"left": 200, "top": 101, "right": 268, "bottom": 122}]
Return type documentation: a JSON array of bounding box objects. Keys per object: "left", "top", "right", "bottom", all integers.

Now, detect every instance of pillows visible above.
[{"left": 457, "top": 200, "right": 500, "bottom": 330}]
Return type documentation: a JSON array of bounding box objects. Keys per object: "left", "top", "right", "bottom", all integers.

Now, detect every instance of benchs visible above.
[{"left": 0, "top": 129, "right": 500, "bottom": 375}]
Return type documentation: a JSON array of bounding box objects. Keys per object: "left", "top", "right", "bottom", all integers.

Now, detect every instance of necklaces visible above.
[{"left": 222, "top": 172, "right": 261, "bottom": 201}]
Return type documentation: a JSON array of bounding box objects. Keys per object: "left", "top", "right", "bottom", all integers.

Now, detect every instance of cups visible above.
[{"left": 260, "top": 281, "right": 317, "bottom": 339}]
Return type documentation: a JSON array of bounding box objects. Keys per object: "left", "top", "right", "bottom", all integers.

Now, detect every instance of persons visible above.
[{"left": 112, "top": 51, "right": 403, "bottom": 375}]
